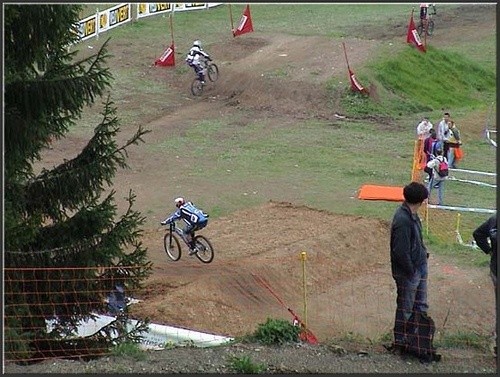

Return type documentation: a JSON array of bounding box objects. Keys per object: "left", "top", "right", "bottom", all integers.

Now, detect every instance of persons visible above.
[
  {"left": 185, "top": 39, "right": 213, "bottom": 83},
  {"left": 390, "top": 182, "right": 429, "bottom": 343},
  {"left": 161, "top": 197, "right": 209, "bottom": 255},
  {"left": 417, "top": 112, "right": 462, "bottom": 169},
  {"left": 426, "top": 148, "right": 447, "bottom": 205},
  {"left": 420, "top": 4, "right": 436, "bottom": 31},
  {"left": 472, "top": 215, "right": 498, "bottom": 358}
]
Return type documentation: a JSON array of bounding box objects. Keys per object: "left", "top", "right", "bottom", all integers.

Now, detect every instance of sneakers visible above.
[{"left": 188, "top": 247, "right": 198, "bottom": 255}]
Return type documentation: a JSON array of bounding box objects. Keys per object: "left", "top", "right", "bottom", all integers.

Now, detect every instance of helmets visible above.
[
  {"left": 175, "top": 197, "right": 185, "bottom": 207},
  {"left": 193, "top": 39, "right": 201, "bottom": 48}
]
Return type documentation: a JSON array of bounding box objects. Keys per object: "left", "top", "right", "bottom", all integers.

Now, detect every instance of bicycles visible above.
[
  {"left": 191, "top": 59, "right": 219, "bottom": 97},
  {"left": 417, "top": 12, "right": 436, "bottom": 38},
  {"left": 160, "top": 221, "right": 214, "bottom": 263}
]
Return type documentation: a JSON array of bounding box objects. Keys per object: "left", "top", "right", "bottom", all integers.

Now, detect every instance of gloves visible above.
[{"left": 161, "top": 220, "right": 166, "bottom": 225}]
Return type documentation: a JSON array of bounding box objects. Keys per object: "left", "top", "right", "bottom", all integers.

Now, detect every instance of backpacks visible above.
[
  {"left": 433, "top": 156, "right": 448, "bottom": 177},
  {"left": 398, "top": 309, "right": 435, "bottom": 362}
]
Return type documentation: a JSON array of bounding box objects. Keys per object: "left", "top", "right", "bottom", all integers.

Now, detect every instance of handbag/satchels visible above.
[{"left": 454, "top": 147, "right": 461, "bottom": 159}]
